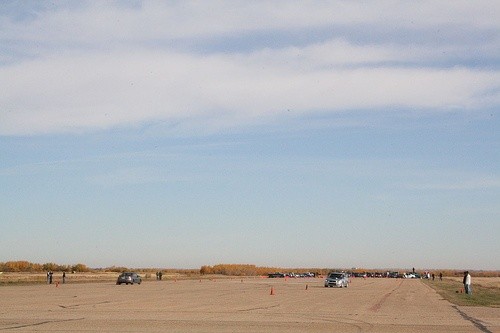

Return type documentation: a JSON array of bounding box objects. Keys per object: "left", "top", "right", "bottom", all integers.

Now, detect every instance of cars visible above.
[
  {"left": 294, "top": 271, "right": 315, "bottom": 277},
  {"left": 117, "top": 272, "right": 142, "bottom": 285},
  {"left": 402, "top": 272, "right": 421, "bottom": 278},
  {"left": 324, "top": 272, "right": 349, "bottom": 288},
  {"left": 390, "top": 271, "right": 398, "bottom": 278}
]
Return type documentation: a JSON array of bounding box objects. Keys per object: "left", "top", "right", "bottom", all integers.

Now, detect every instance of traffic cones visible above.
[
  {"left": 270, "top": 287, "right": 274, "bottom": 295},
  {"left": 305, "top": 284, "right": 309, "bottom": 290}
]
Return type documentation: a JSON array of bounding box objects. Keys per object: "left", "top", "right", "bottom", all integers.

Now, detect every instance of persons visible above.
[
  {"left": 427, "top": 272, "right": 434, "bottom": 281},
  {"left": 438, "top": 271, "right": 443, "bottom": 281},
  {"left": 412, "top": 267, "right": 415, "bottom": 272},
  {"left": 462, "top": 270, "right": 473, "bottom": 296}
]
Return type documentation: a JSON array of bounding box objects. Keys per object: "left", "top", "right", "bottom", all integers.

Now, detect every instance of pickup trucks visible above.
[{"left": 268, "top": 272, "right": 286, "bottom": 278}]
